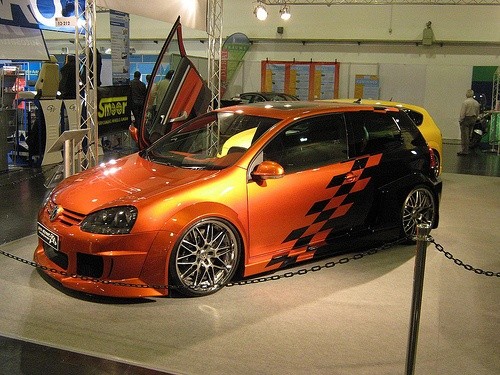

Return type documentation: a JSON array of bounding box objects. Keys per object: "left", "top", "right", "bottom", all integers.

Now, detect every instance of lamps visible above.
[
  {"left": 254, "top": 5, "right": 268, "bottom": 20},
  {"left": 281, "top": 8, "right": 290, "bottom": 22}
]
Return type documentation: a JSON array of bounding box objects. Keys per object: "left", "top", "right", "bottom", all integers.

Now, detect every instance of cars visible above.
[
  {"left": 32, "top": 16, "right": 443, "bottom": 298},
  {"left": 240, "top": 91, "right": 302, "bottom": 103},
  {"left": 314, "top": 98, "right": 443, "bottom": 197}
]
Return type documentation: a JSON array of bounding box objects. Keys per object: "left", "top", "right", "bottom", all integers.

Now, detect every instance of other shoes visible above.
[{"left": 457, "top": 149, "right": 477, "bottom": 157}]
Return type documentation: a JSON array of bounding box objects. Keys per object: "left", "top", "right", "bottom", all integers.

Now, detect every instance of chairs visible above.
[
  {"left": 19, "top": 55, "right": 59, "bottom": 100},
  {"left": 57, "top": 55, "right": 81, "bottom": 100}
]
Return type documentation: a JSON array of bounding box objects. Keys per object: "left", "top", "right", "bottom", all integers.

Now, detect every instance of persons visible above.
[
  {"left": 128, "top": 69, "right": 176, "bottom": 137},
  {"left": 459, "top": 89, "right": 479, "bottom": 155}
]
